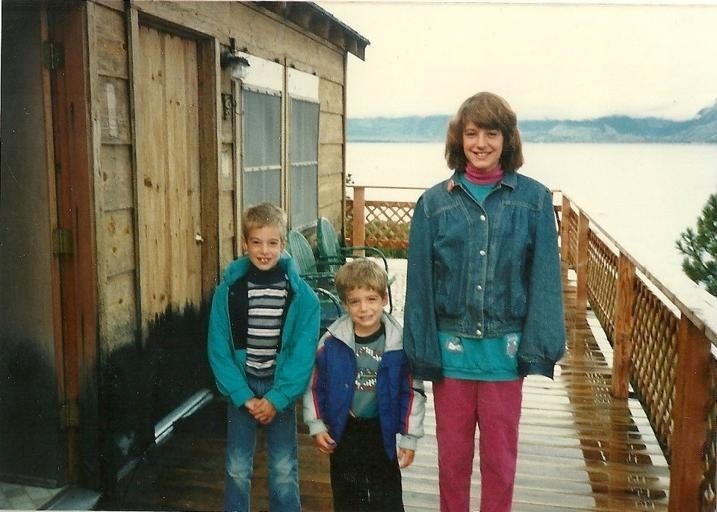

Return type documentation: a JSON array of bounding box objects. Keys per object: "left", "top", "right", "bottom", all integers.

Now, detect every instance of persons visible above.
[
  {"left": 206, "top": 201, "right": 321, "bottom": 512},
  {"left": 402, "top": 91, "right": 566, "bottom": 512},
  {"left": 303, "top": 258, "right": 426, "bottom": 512}
]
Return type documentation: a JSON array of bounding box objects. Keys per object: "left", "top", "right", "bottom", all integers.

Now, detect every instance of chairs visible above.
[
  {"left": 287, "top": 230, "right": 345, "bottom": 328},
  {"left": 316, "top": 216, "right": 394, "bottom": 315}
]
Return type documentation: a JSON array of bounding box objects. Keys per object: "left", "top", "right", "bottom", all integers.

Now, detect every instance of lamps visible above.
[{"left": 220, "top": 48, "right": 249, "bottom": 79}]
